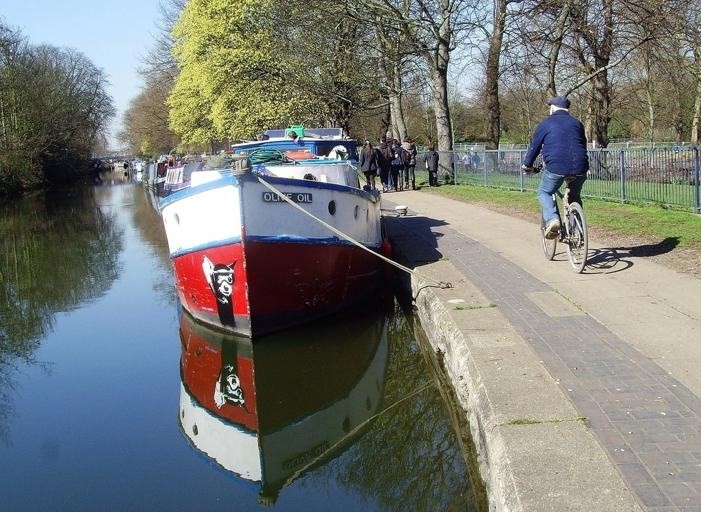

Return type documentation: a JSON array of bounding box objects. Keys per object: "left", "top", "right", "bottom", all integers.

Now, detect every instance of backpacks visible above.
[{"left": 401, "top": 149, "right": 411, "bottom": 165}]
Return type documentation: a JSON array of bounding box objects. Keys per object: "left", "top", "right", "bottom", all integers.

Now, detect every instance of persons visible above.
[
  {"left": 522, "top": 94, "right": 591, "bottom": 247},
  {"left": 360, "top": 130, "right": 481, "bottom": 194}
]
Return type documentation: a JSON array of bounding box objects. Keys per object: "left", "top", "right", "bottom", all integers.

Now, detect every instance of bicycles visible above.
[{"left": 524, "top": 165, "right": 590, "bottom": 275}]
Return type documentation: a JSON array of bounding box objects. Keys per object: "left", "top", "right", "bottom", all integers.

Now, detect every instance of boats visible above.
[
  {"left": 174, "top": 306, "right": 393, "bottom": 508},
  {"left": 124, "top": 127, "right": 362, "bottom": 218},
  {"left": 154, "top": 129, "right": 392, "bottom": 342}
]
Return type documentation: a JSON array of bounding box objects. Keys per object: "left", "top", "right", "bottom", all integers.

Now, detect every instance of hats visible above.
[{"left": 548, "top": 96, "right": 570, "bottom": 108}]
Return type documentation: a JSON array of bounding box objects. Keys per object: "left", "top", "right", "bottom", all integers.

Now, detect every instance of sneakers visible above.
[
  {"left": 544, "top": 221, "right": 560, "bottom": 239},
  {"left": 383, "top": 180, "right": 415, "bottom": 192}
]
